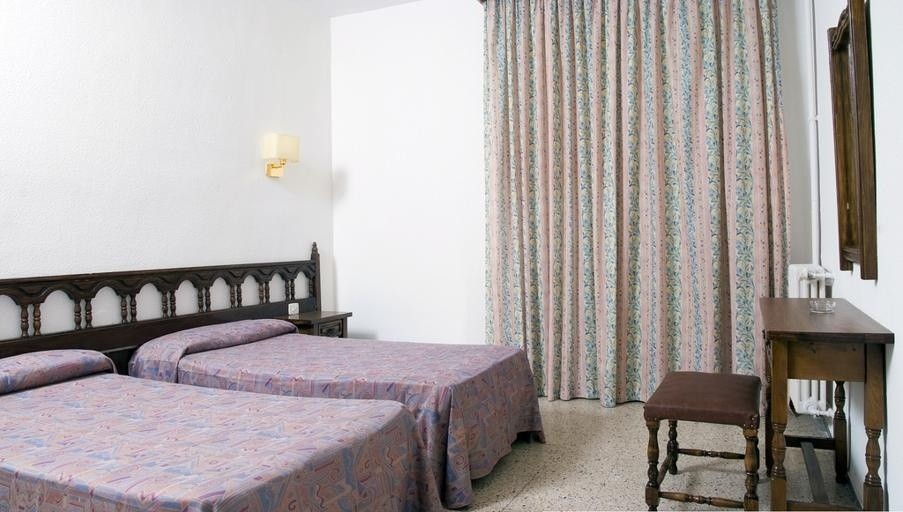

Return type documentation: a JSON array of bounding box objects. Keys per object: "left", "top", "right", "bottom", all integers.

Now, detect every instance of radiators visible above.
[{"left": 787, "top": 262, "right": 838, "bottom": 415}]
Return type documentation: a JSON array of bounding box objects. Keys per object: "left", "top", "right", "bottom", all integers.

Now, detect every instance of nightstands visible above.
[{"left": 274, "top": 311, "right": 351, "bottom": 339}]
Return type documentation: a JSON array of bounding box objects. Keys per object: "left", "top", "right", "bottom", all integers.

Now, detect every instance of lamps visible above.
[{"left": 258, "top": 130, "right": 301, "bottom": 180}]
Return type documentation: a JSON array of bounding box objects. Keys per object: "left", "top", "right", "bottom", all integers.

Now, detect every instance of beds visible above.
[
  {"left": 128, "top": 244, "right": 547, "bottom": 511},
  {"left": 0, "top": 242, "right": 443, "bottom": 512}
]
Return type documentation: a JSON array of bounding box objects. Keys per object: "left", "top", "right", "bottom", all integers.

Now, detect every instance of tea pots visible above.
[{"left": 795, "top": 263, "right": 836, "bottom": 315}]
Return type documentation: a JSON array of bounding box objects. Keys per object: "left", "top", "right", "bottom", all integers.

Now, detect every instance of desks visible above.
[{"left": 758, "top": 298, "right": 895, "bottom": 510}]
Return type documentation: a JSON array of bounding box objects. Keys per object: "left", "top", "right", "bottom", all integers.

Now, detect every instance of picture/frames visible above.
[{"left": 823, "top": 1, "right": 880, "bottom": 280}]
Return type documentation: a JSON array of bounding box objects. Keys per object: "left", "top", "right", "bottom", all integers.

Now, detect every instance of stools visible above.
[{"left": 635, "top": 370, "right": 764, "bottom": 511}]
